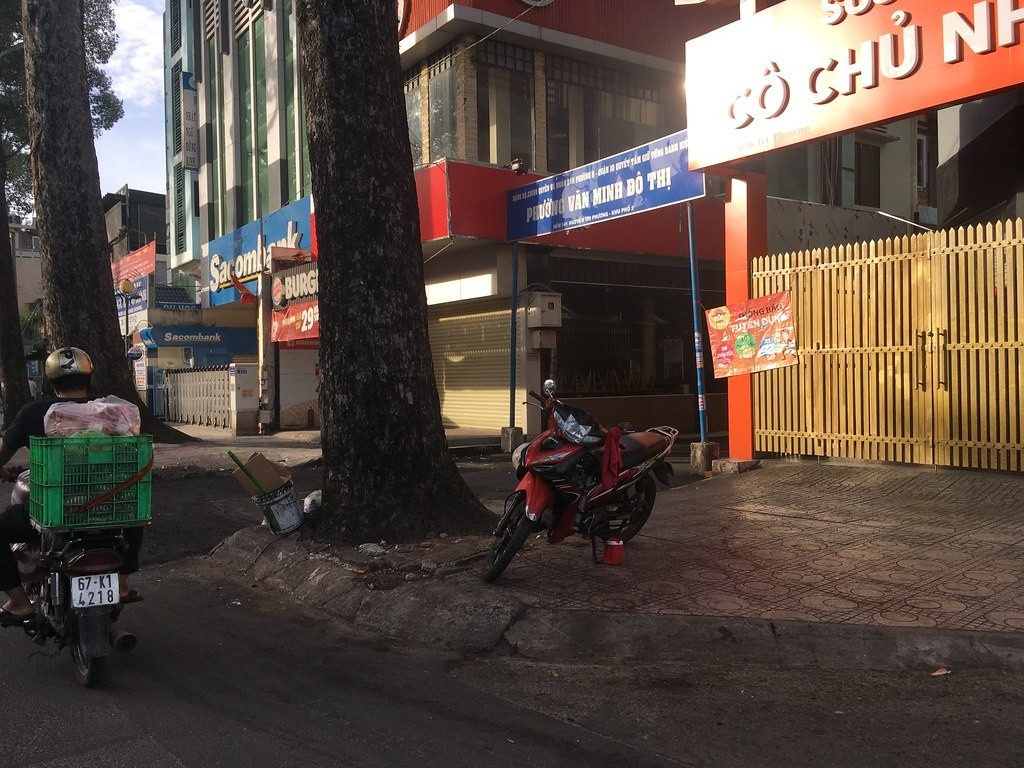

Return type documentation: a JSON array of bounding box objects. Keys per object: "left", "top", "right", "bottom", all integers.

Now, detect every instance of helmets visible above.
[
  {"left": 45, "top": 346, "right": 94, "bottom": 379},
  {"left": 512, "top": 442, "right": 531, "bottom": 481}
]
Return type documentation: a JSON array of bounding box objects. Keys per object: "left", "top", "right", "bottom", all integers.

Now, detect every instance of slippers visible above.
[
  {"left": 0, "top": 606, "right": 37, "bottom": 627},
  {"left": 119, "top": 589, "right": 143, "bottom": 604}
]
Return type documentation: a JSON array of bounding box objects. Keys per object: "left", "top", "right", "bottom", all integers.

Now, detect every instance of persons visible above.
[{"left": 0, "top": 347, "right": 143, "bottom": 627}]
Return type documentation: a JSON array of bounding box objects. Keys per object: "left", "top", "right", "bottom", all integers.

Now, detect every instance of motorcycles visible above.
[
  {"left": 0, "top": 464, "right": 137, "bottom": 689},
  {"left": 482, "top": 379, "right": 679, "bottom": 582}
]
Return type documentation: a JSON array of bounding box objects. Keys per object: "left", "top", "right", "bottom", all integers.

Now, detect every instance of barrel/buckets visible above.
[
  {"left": 251, "top": 478, "right": 305, "bottom": 536},
  {"left": 604, "top": 538, "right": 623, "bottom": 565}
]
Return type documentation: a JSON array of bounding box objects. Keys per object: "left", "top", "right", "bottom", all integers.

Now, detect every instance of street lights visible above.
[{"left": 115, "top": 278, "right": 142, "bottom": 359}]
